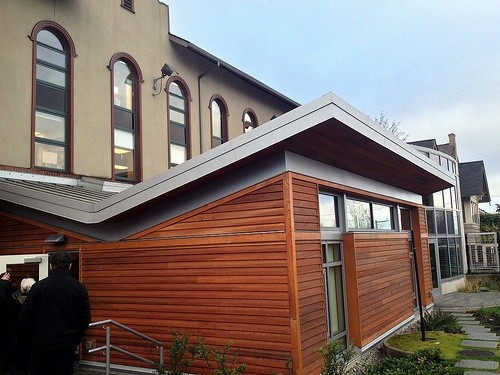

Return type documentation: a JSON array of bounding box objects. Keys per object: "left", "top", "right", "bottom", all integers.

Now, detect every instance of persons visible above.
[
  {"left": 17, "top": 277, "right": 37, "bottom": 304},
  {"left": 19, "top": 250, "right": 92, "bottom": 375},
  {"left": 0, "top": 272, "right": 22, "bottom": 375}
]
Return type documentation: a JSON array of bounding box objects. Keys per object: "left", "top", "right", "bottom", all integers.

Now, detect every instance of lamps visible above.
[{"left": 152, "top": 62, "right": 176, "bottom": 96}]
